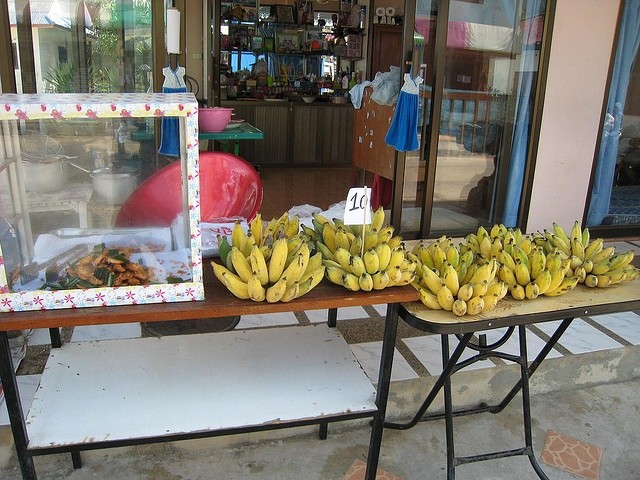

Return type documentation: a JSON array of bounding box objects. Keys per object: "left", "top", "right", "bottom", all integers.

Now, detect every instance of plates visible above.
[{"left": 225, "top": 118, "right": 245, "bottom": 129}]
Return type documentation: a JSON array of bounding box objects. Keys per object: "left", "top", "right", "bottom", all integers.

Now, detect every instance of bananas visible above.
[
  {"left": 531, "top": 221, "right": 640, "bottom": 287},
  {"left": 459, "top": 224, "right": 580, "bottom": 302},
  {"left": 406, "top": 235, "right": 509, "bottom": 316},
  {"left": 211, "top": 212, "right": 326, "bottom": 304},
  {"left": 301, "top": 206, "right": 417, "bottom": 291}
]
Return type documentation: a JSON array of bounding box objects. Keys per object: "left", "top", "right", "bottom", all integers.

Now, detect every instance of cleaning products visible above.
[{"left": 349, "top": 71, "right": 357, "bottom": 90}]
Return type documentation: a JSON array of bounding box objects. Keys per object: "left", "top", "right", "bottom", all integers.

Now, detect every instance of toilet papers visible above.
[
  {"left": 386, "top": 7, "right": 395, "bottom": 17},
  {"left": 375, "top": 7, "right": 386, "bottom": 18}
]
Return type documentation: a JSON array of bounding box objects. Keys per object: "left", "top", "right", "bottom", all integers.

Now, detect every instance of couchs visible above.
[{"left": 404, "top": 135, "right": 495, "bottom": 203}]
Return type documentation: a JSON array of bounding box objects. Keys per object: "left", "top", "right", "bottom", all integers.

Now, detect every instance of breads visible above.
[{"left": 66, "top": 246, "right": 149, "bottom": 290}]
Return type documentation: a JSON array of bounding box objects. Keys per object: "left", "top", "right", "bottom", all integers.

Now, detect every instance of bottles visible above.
[{"left": 342, "top": 75, "right": 349, "bottom": 89}]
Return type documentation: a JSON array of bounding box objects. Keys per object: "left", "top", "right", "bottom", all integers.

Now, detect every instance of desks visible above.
[
  {"left": 0, "top": 256, "right": 422, "bottom": 480},
  {"left": 131, "top": 123, "right": 265, "bottom": 179},
  {"left": 369, "top": 259, "right": 640, "bottom": 480},
  {"left": 26, "top": 182, "right": 95, "bottom": 228}
]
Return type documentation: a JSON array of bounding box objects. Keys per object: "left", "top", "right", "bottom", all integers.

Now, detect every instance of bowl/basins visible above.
[
  {"left": 302, "top": 95, "right": 316, "bottom": 103},
  {"left": 199, "top": 106, "right": 235, "bottom": 131}
]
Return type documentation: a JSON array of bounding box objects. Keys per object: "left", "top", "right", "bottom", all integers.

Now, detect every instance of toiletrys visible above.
[{"left": 342, "top": 72, "right": 348, "bottom": 89}]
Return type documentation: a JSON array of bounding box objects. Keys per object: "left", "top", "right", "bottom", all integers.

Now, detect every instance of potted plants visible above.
[{"left": 90, "top": 69, "right": 112, "bottom": 131}]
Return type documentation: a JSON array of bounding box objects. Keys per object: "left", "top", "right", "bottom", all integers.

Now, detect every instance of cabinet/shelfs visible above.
[
  {"left": 352, "top": 88, "right": 400, "bottom": 188},
  {"left": 212, "top": 0, "right": 370, "bottom": 105}
]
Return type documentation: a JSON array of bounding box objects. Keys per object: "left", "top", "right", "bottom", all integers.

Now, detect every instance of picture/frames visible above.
[
  {"left": 275, "top": 32, "right": 301, "bottom": 52},
  {"left": 339, "top": 0, "right": 353, "bottom": 13},
  {"left": 275, "top": 4, "right": 296, "bottom": 23},
  {"left": 250, "top": 35, "right": 264, "bottom": 52},
  {"left": 263, "top": 37, "right": 275, "bottom": 52},
  {"left": 278, "top": 54, "right": 305, "bottom": 76}
]
